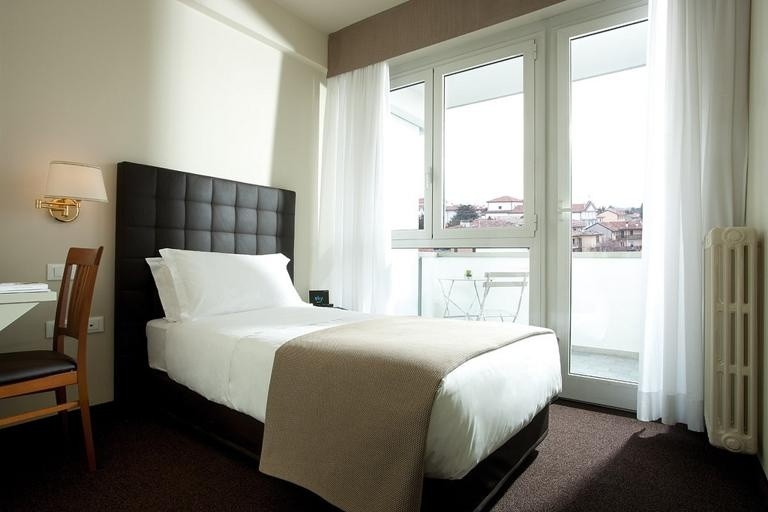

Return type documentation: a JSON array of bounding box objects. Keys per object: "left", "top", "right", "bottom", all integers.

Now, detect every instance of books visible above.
[{"left": 0, "top": 281, "right": 51, "bottom": 293}]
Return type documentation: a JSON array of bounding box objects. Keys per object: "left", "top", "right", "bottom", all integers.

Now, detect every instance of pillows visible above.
[
  {"left": 156, "top": 246, "right": 309, "bottom": 323},
  {"left": 143, "top": 256, "right": 180, "bottom": 323}
]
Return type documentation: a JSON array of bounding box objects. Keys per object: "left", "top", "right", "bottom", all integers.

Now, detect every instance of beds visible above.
[{"left": 113, "top": 158, "right": 565, "bottom": 511}]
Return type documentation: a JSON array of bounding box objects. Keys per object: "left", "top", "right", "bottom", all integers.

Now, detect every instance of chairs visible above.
[
  {"left": 475, "top": 269, "right": 528, "bottom": 323},
  {"left": 0, "top": 244, "right": 103, "bottom": 471}
]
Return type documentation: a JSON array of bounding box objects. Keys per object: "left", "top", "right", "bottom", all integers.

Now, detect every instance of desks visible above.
[{"left": 439, "top": 276, "right": 490, "bottom": 322}]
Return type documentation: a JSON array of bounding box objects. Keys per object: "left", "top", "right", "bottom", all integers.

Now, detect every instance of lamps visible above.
[{"left": 32, "top": 160, "right": 111, "bottom": 224}]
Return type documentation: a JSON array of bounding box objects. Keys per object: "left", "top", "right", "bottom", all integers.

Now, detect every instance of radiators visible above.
[{"left": 702, "top": 223, "right": 763, "bottom": 456}]
